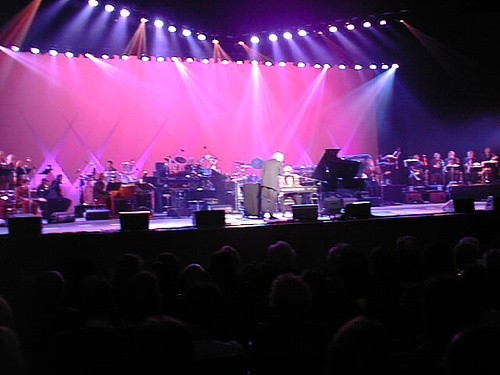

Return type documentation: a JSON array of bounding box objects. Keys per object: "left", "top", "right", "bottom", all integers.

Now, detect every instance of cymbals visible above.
[
  {"left": 164, "top": 158, "right": 176, "bottom": 163},
  {"left": 234, "top": 161, "right": 252, "bottom": 165},
  {"left": 176, "top": 157, "right": 187, "bottom": 164}
]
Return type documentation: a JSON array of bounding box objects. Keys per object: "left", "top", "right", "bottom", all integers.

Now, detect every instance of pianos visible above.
[{"left": 299, "top": 148, "right": 369, "bottom": 216}]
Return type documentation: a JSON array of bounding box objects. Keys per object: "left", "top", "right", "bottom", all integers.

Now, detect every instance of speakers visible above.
[
  {"left": 344, "top": 201, "right": 371, "bottom": 218},
  {"left": 292, "top": 204, "right": 318, "bottom": 221},
  {"left": 118, "top": 211, "right": 150, "bottom": 230},
  {"left": 7, "top": 214, "right": 43, "bottom": 234},
  {"left": 192, "top": 210, "right": 226, "bottom": 229}
]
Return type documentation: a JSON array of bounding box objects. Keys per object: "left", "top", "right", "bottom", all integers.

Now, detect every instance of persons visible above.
[
  {"left": 105, "top": 159, "right": 117, "bottom": 178},
  {"left": 0, "top": 151, "right": 71, "bottom": 220},
  {"left": 93, "top": 173, "right": 112, "bottom": 209},
  {"left": 260, "top": 151, "right": 284, "bottom": 219},
  {"left": 131, "top": 160, "right": 138, "bottom": 171},
  {"left": 380, "top": 147, "right": 500, "bottom": 187},
  {"left": 0, "top": 236, "right": 500, "bottom": 375}
]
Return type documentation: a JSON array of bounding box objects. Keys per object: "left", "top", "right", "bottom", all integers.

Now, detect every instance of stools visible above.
[{"left": 93, "top": 198, "right": 107, "bottom": 209}]
